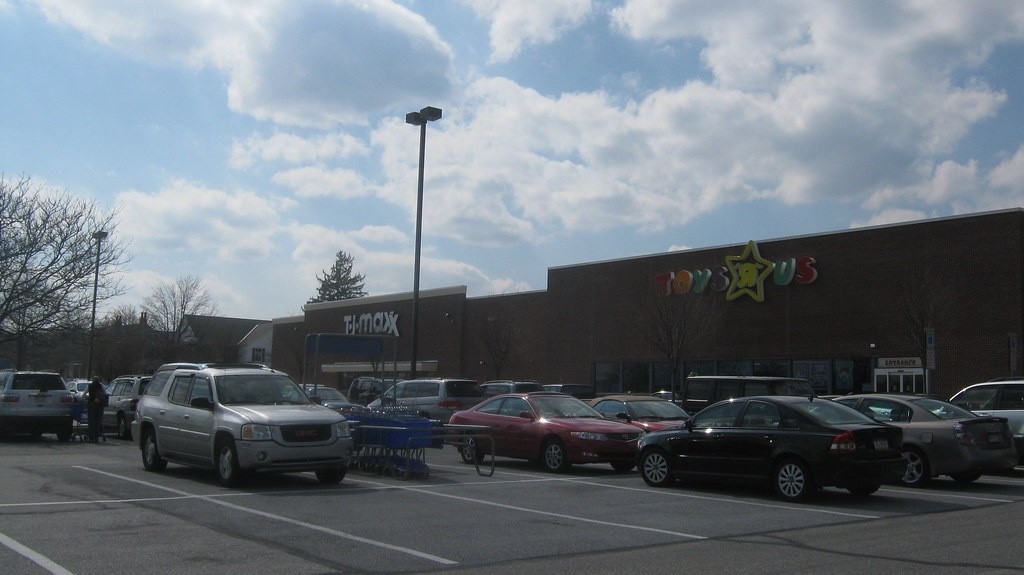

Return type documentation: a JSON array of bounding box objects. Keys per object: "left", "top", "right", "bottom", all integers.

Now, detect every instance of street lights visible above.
[
  {"left": 405, "top": 106, "right": 443, "bottom": 379},
  {"left": 86, "top": 230, "right": 109, "bottom": 379}
]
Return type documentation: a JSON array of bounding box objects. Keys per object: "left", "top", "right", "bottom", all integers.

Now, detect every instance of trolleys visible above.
[
  {"left": 71, "top": 419, "right": 105, "bottom": 442},
  {"left": 338, "top": 404, "right": 430, "bottom": 481}
]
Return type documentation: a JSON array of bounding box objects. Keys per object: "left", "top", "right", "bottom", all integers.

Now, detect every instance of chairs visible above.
[
  {"left": 889, "top": 409, "right": 908, "bottom": 421},
  {"left": 748, "top": 408, "right": 767, "bottom": 426}
]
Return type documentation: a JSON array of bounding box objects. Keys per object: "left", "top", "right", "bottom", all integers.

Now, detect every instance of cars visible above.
[
  {"left": 367, "top": 378, "right": 485, "bottom": 423},
  {"left": 574, "top": 395, "right": 692, "bottom": 434},
  {"left": 634, "top": 396, "right": 908, "bottom": 502},
  {"left": 68, "top": 379, "right": 92, "bottom": 406},
  {"left": 445, "top": 390, "right": 649, "bottom": 475},
  {"left": 832, "top": 392, "right": 1016, "bottom": 488}
]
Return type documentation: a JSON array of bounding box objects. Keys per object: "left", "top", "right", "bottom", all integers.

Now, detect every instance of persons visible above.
[
  {"left": 81, "top": 376, "right": 106, "bottom": 443},
  {"left": 773, "top": 382, "right": 783, "bottom": 394},
  {"left": 349, "top": 379, "right": 366, "bottom": 403}
]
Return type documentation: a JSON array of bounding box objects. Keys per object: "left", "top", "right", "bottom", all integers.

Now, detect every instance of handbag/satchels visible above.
[
  {"left": 94, "top": 384, "right": 109, "bottom": 406},
  {"left": 71, "top": 399, "right": 82, "bottom": 422}
]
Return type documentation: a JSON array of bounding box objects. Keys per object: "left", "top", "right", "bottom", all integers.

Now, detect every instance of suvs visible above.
[
  {"left": 0, "top": 368, "right": 75, "bottom": 442},
  {"left": 281, "top": 383, "right": 365, "bottom": 427},
  {"left": 480, "top": 379, "right": 546, "bottom": 400},
  {"left": 348, "top": 376, "right": 402, "bottom": 405},
  {"left": 101, "top": 374, "right": 154, "bottom": 441},
  {"left": 130, "top": 361, "right": 355, "bottom": 486},
  {"left": 931, "top": 376, "right": 1024, "bottom": 466}
]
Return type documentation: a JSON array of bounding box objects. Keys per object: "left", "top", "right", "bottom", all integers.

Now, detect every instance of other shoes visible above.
[{"left": 90, "top": 439, "right": 98, "bottom": 443}]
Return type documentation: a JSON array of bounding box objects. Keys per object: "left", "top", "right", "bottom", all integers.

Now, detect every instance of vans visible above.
[{"left": 543, "top": 383, "right": 596, "bottom": 398}]
